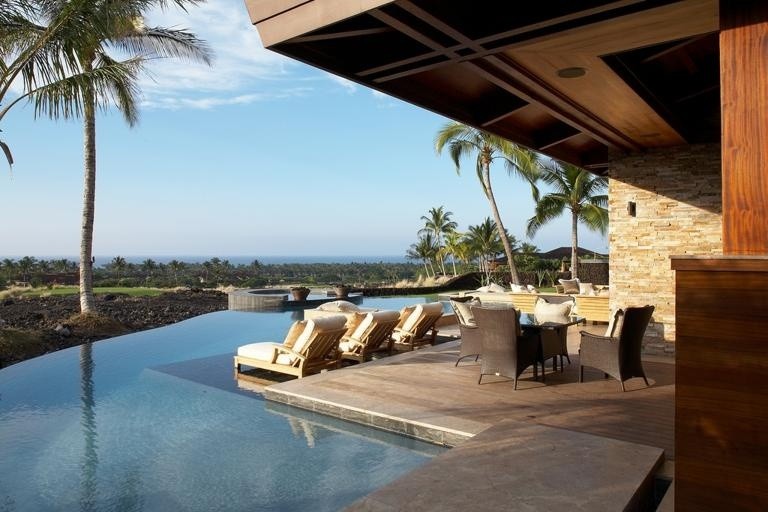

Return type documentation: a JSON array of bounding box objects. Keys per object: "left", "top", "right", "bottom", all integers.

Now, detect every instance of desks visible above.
[{"left": 595, "top": 284, "right": 608, "bottom": 293}]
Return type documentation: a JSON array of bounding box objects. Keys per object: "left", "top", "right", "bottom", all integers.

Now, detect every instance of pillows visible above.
[{"left": 477, "top": 277, "right": 595, "bottom": 295}]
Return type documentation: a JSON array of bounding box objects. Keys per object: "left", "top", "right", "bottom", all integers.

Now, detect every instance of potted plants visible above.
[
  {"left": 331, "top": 283, "right": 352, "bottom": 296},
  {"left": 291, "top": 284, "right": 311, "bottom": 303}
]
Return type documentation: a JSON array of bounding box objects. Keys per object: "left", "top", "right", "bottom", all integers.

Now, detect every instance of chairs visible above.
[
  {"left": 450, "top": 295, "right": 654, "bottom": 393},
  {"left": 229, "top": 301, "right": 444, "bottom": 388}
]
